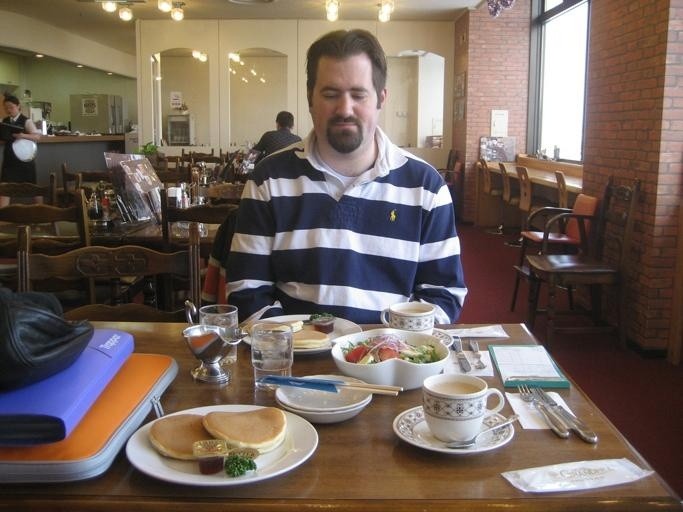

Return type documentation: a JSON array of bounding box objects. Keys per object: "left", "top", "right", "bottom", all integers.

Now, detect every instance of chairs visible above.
[
  {"left": 499, "top": 163, "right": 551, "bottom": 248},
  {"left": 436, "top": 160, "right": 462, "bottom": 187},
  {"left": 160, "top": 152, "right": 179, "bottom": 189},
  {"left": 0, "top": 173, "right": 58, "bottom": 234},
  {"left": 14, "top": 221, "right": 208, "bottom": 323},
  {"left": 445, "top": 148, "right": 458, "bottom": 181},
  {"left": 526, "top": 174, "right": 641, "bottom": 355},
  {"left": 515, "top": 166, "right": 557, "bottom": 223},
  {"left": 555, "top": 171, "right": 568, "bottom": 208},
  {"left": 480, "top": 159, "right": 520, "bottom": 236},
  {"left": 159, "top": 189, "right": 239, "bottom": 246},
  {"left": 62, "top": 163, "right": 81, "bottom": 201},
  {"left": 1, "top": 189, "right": 158, "bottom": 309},
  {"left": 181, "top": 148, "right": 249, "bottom": 184},
  {"left": 510, "top": 192, "right": 602, "bottom": 312}
]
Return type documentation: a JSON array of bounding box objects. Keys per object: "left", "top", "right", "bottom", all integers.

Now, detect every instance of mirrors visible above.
[
  {"left": 230, "top": 48, "right": 288, "bottom": 146},
  {"left": 152, "top": 48, "right": 210, "bottom": 147}
]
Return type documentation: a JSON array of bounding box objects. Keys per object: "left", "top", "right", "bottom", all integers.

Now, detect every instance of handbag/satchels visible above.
[
  {"left": 0, "top": 289, "right": 93, "bottom": 391},
  {"left": 0, "top": 354, "right": 179, "bottom": 483}
]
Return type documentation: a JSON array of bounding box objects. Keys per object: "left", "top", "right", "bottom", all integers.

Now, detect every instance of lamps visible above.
[
  {"left": 171, "top": 1, "right": 185, "bottom": 22},
  {"left": 118, "top": 1, "right": 134, "bottom": 22}
]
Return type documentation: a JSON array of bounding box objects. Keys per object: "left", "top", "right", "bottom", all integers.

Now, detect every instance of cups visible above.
[
  {"left": 199, "top": 304, "right": 237, "bottom": 364},
  {"left": 250, "top": 321, "right": 295, "bottom": 389},
  {"left": 380, "top": 302, "right": 436, "bottom": 333},
  {"left": 422, "top": 371, "right": 506, "bottom": 445}
]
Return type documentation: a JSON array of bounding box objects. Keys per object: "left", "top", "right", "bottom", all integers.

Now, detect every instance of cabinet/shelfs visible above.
[
  {"left": 167, "top": 115, "right": 196, "bottom": 145},
  {"left": 70, "top": 94, "right": 124, "bottom": 134},
  {"left": 0, "top": 135, "right": 125, "bottom": 185}
]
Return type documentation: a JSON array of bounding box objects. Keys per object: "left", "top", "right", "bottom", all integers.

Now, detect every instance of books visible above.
[{"left": 488, "top": 344, "right": 571, "bottom": 390}]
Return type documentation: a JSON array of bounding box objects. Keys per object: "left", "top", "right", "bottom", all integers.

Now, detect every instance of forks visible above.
[
  {"left": 469, "top": 338, "right": 488, "bottom": 369},
  {"left": 530, "top": 386, "right": 597, "bottom": 444},
  {"left": 517, "top": 382, "right": 572, "bottom": 438}
]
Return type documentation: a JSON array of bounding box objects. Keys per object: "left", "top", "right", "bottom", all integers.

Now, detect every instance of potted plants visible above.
[{"left": 134, "top": 142, "right": 167, "bottom": 177}]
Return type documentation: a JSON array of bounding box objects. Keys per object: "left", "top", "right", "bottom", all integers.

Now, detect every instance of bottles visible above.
[
  {"left": 102, "top": 191, "right": 110, "bottom": 222},
  {"left": 192, "top": 196, "right": 208, "bottom": 237},
  {"left": 177, "top": 182, "right": 192, "bottom": 228},
  {"left": 87, "top": 192, "right": 102, "bottom": 220}
]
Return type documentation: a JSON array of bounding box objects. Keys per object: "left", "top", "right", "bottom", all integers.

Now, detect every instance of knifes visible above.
[{"left": 452, "top": 335, "right": 470, "bottom": 372}]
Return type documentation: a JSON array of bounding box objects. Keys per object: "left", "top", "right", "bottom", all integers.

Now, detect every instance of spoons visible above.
[{"left": 447, "top": 414, "right": 521, "bottom": 449}]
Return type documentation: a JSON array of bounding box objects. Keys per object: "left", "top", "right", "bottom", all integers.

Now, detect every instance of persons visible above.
[
  {"left": 226, "top": 28, "right": 468, "bottom": 327},
  {"left": 0, "top": 95, "right": 43, "bottom": 206},
  {"left": 247, "top": 110, "right": 301, "bottom": 164}
]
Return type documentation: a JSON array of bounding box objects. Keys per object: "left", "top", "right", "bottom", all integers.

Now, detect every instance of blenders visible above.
[{"left": 27, "top": 100, "right": 52, "bottom": 136}]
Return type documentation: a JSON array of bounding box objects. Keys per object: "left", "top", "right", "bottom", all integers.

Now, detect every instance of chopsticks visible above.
[{"left": 257, "top": 374, "right": 405, "bottom": 398}]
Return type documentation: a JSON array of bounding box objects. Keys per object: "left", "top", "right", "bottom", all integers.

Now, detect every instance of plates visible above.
[
  {"left": 125, "top": 403, "right": 319, "bottom": 487},
  {"left": 429, "top": 329, "right": 453, "bottom": 350},
  {"left": 238, "top": 313, "right": 363, "bottom": 357},
  {"left": 391, "top": 404, "right": 517, "bottom": 457}
]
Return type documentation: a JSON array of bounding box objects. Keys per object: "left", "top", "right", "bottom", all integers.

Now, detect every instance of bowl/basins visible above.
[
  {"left": 275, "top": 374, "right": 374, "bottom": 424},
  {"left": 331, "top": 326, "right": 451, "bottom": 392}
]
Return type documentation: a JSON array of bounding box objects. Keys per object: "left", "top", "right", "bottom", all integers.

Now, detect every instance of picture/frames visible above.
[
  {"left": 453, "top": 97, "right": 466, "bottom": 122},
  {"left": 454, "top": 71, "right": 466, "bottom": 98},
  {"left": 480, "top": 136, "right": 516, "bottom": 163}
]
